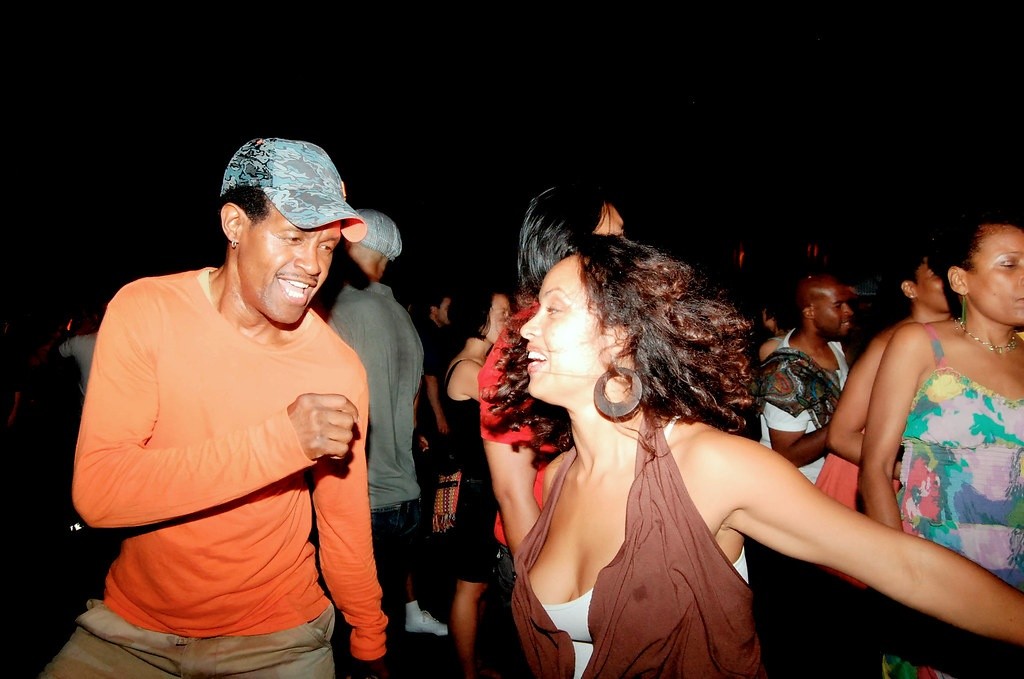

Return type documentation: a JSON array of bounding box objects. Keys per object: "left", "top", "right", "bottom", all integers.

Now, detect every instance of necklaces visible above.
[{"left": 956, "top": 318, "right": 1021, "bottom": 356}]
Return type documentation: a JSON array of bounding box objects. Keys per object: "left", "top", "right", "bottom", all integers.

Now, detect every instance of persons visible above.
[
  {"left": 40, "top": 137, "right": 387, "bottom": 679},
  {"left": 484, "top": 188, "right": 625, "bottom": 568},
  {"left": 0, "top": 211, "right": 952, "bottom": 679},
  {"left": 512, "top": 237, "right": 1023, "bottom": 679},
  {"left": 859, "top": 204, "right": 1023, "bottom": 679}
]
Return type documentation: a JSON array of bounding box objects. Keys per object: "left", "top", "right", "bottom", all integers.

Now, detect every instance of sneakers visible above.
[{"left": 404, "top": 599, "right": 450, "bottom": 637}]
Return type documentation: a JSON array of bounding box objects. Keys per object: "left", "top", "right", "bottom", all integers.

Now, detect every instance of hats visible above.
[
  {"left": 220, "top": 138, "right": 369, "bottom": 243},
  {"left": 356, "top": 210, "right": 402, "bottom": 262}
]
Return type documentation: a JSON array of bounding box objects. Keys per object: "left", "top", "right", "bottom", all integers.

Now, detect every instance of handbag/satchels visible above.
[{"left": 431, "top": 469, "right": 462, "bottom": 533}]
